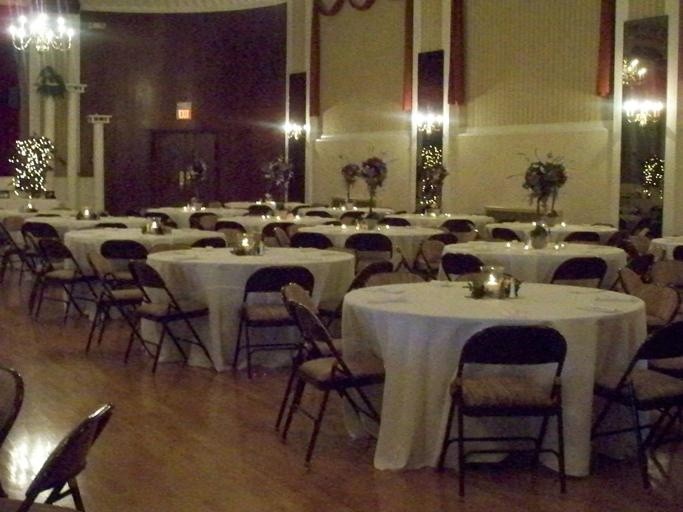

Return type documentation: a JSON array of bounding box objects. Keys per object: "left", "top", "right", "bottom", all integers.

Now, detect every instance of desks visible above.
[{"left": 340, "top": 280, "right": 648, "bottom": 476}]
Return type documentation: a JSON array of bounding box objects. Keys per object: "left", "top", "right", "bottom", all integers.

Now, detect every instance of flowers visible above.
[
  {"left": 336, "top": 154, "right": 360, "bottom": 187},
  {"left": 355, "top": 144, "right": 395, "bottom": 189},
  {"left": 503, "top": 146, "right": 570, "bottom": 218}
]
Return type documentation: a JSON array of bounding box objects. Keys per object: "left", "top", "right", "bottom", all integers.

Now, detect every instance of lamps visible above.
[
  {"left": 2, "top": 0, "right": 78, "bottom": 57},
  {"left": 619, "top": 53, "right": 645, "bottom": 87},
  {"left": 618, "top": 96, "right": 665, "bottom": 128},
  {"left": 414, "top": 106, "right": 441, "bottom": 138}
]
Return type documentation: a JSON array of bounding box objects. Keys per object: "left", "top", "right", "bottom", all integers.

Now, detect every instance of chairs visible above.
[
  {"left": 436, "top": 324, "right": 569, "bottom": 500},
  {"left": 0, "top": 366, "right": 26, "bottom": 498},
  {"left": 592, "top": 322, "right": 682, "bottom": 490},
  {"left": 0, "top": 198, "right": 683, "bottom": 377},
  {"left": 0, "top": 402, "right": 116, "bottom": 512},
  {"left": 274, "top": 283, "right": 387, "bottom": 463}
]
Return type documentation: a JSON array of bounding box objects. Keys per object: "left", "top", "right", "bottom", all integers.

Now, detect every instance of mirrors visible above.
[
  {"left": 617, "top": 14, "right": 669, "bottom": 241},
  {"left": 285, "top": 70, "right": 307, "bottom": 203},
  {"left": 415, "top": 49, "right": 441, "bottom": 214}
]
